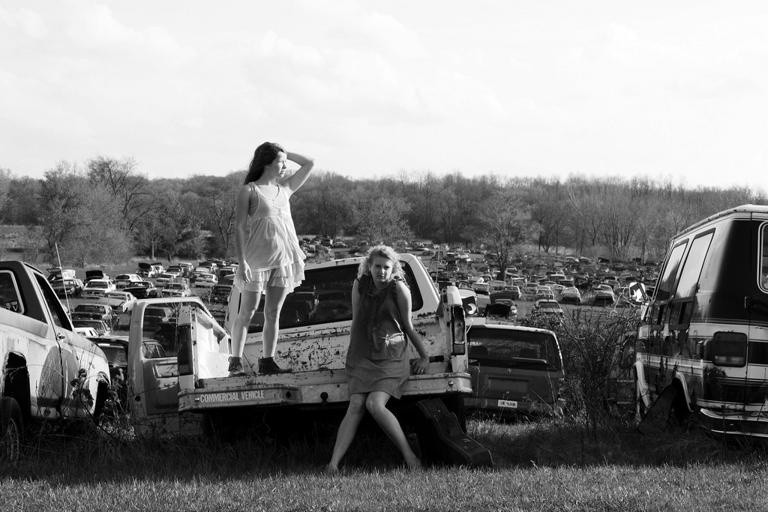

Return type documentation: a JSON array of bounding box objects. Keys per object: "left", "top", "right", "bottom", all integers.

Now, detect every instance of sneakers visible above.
[
  {"left": 228, "top": 356, "right": 246, "bottom": 377},
  {"left": 258, "top": 358, "right": 293, "bottom": 373}
]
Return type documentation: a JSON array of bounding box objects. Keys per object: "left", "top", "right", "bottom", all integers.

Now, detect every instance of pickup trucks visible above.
[{"left": 0, "top": 258, "right": 114, "bottom": 476}]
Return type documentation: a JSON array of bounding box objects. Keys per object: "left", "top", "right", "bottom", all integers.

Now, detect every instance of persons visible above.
[
  {"left": 321, "top": 245, "right": 428, "bottom": 471},
  {"left": 227, "top": 141, "right": 314, "bottom": 379}
]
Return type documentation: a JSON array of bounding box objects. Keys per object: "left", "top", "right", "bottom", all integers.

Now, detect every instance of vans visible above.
[{"left": 620, "top": 199, "right": 767, "bottom": 462}]
